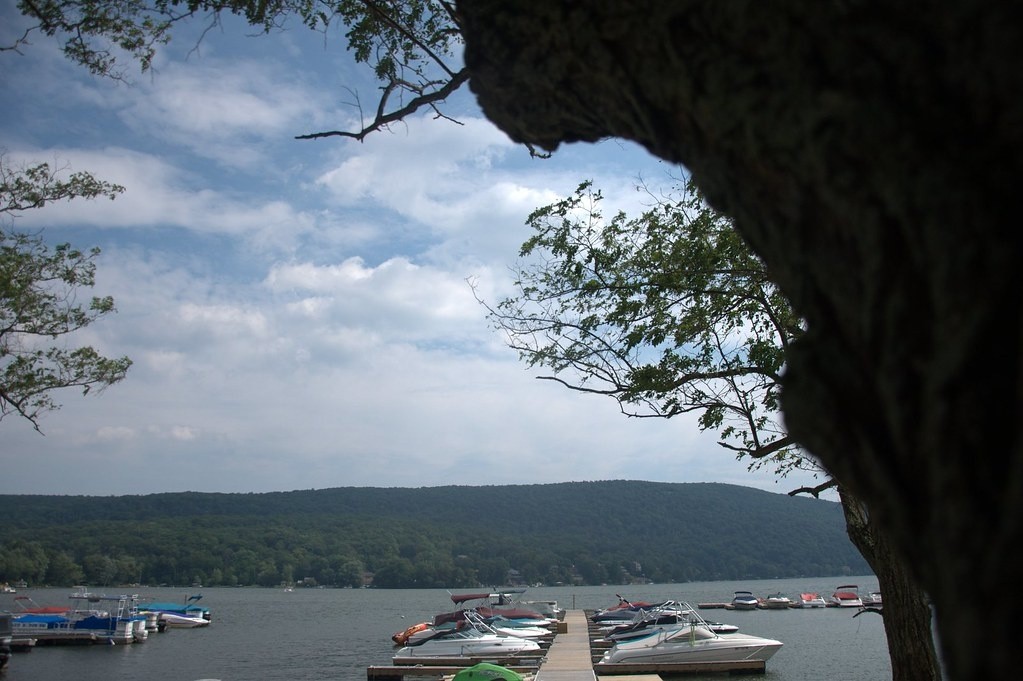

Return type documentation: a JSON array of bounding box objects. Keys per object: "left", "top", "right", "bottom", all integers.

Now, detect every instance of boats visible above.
[
  {"left": 391, "top": 588, "right": 563, "bottom": 661},
  {"left": 588, "top": 584, "right": 883, "bottom": 676},
  {"left": 0, "top": 578, "right": 211, "bottom": 664}
]
